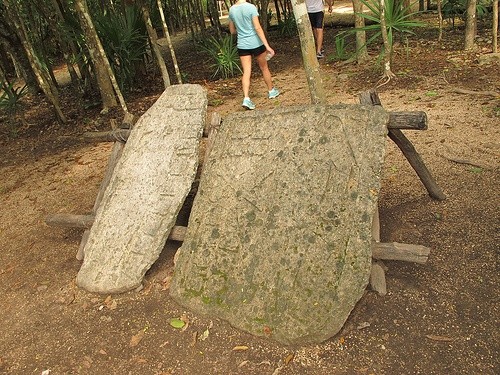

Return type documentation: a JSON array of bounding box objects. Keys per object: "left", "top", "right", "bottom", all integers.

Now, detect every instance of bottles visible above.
[{"left": 263, "top": 51, "right": 273, "bottom": 61}]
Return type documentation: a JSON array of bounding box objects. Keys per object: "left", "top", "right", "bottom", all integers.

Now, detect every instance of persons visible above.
[
  {"left": 228, "top": 0, "right": 281, "bottom": 109},
  {"left": 305, "top": 0, "right": 334, "bottom": 58}
]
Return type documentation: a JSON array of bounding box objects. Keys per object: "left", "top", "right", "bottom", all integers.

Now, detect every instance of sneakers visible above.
[
  {"left": 320, "top": 48, "right": 326, "bottom": 53},
  {"left": 268, "top": 87, "right": 280, "bottom": 99},
  {"left": 242, "top": 99, "right": 256, "bottom": 110},
  {"left": 316, "top": 52, "right": 324, "bottom": 58}
]
